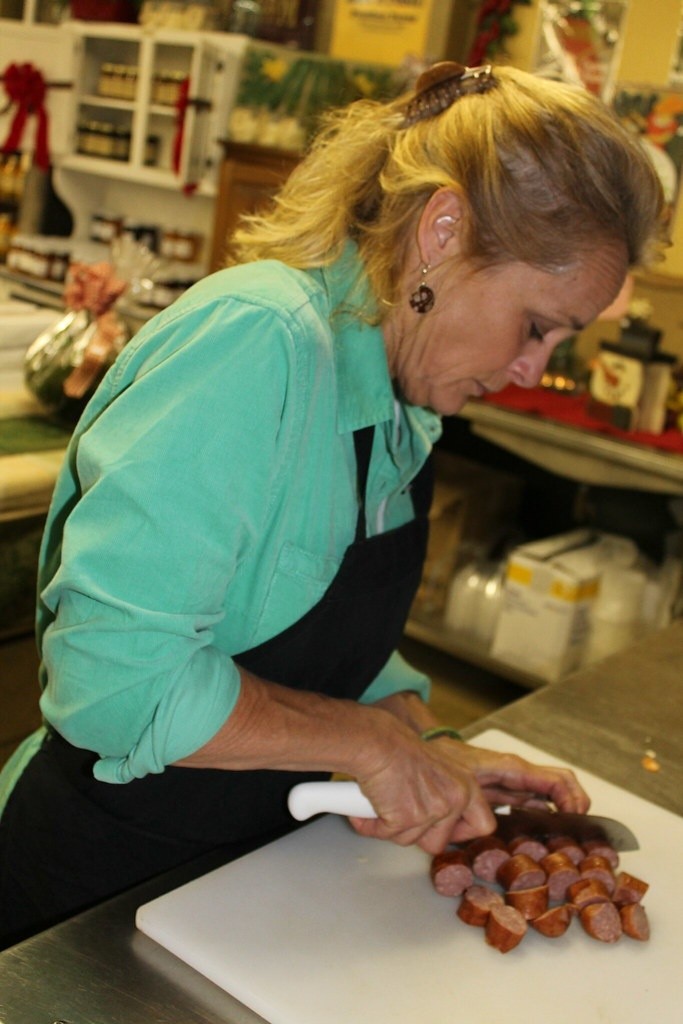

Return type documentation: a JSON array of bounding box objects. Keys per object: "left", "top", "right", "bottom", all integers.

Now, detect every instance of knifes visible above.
[{"left": 287, "top": 782, "right": 641, "bottom": 852}]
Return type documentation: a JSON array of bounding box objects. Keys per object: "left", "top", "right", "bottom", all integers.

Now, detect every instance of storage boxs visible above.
[{"left": 490, "top": 529, "right": 638, "bottom": 684}]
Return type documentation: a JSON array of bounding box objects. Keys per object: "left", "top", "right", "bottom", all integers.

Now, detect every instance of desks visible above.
[{"left": 0, "top": 618, "right": 683, "bottom": 1024}]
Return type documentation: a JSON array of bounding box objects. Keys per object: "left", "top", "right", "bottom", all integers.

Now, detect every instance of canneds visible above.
[
  {"left": 78, "top": 61, "right": 189, "bottom": 166},
  {"left": 5, "top": 232, "right": 196, "bottom": 310},
  {"left": 88, "top": 209, "right": 198, "bottom": 261}
]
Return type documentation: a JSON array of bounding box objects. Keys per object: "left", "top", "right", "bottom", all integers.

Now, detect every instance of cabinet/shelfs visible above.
[{"left": 0, "top": 0, "right": 333, "bottom": 332}]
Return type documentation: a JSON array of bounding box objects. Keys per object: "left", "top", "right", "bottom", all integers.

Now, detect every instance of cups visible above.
[{"left": 590, "top": 568, "right": 643, "bottom": 665}]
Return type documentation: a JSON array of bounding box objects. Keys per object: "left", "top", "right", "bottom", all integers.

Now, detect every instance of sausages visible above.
[{"left": 431, "top": 834, "right": 650, "bottom": 953}]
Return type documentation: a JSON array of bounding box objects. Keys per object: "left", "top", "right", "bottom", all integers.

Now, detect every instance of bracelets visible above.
[{"left": 421, "top": 726, "right": 465, "bottom": 746}]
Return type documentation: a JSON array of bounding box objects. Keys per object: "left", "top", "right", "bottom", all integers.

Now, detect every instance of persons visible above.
[{"left": 0, "top": 62, "right": 663, "bottom": 951}]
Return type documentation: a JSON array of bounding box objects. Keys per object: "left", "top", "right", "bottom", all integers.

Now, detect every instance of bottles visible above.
[
  {"left": 98, "top": 63, "right": 188, "bottom": 107},
  {"left": 91, "top": 209, "right": 201, "bottom": 261},
  {"left": 441, "top": 551, "right": 510, "bottom": 646},
  {"left": 6, "top": 233, "right": 78, "bottom": 278},
  {"left": 77, "top": 119, "right": 160, "bottom": 165}
]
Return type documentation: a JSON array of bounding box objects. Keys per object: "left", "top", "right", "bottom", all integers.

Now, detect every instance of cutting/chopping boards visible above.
[{"left": 133, "top": 729, "right": 683, "bottom": 1024}]
